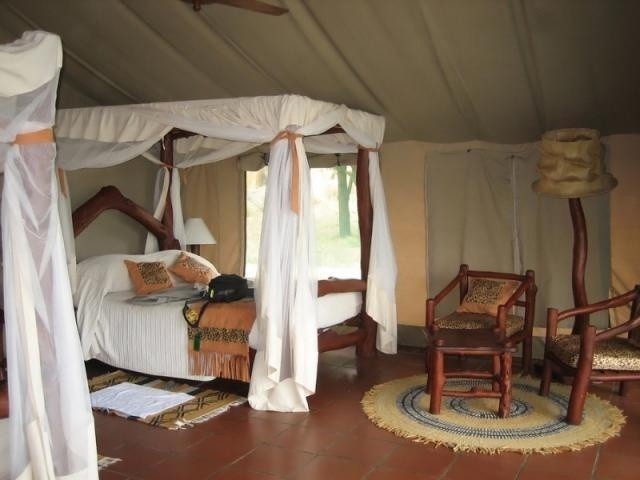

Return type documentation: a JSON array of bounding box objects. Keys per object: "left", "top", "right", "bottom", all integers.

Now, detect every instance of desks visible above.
[{"left": 425, "top": 324, "right": 518, "bottom": 419}]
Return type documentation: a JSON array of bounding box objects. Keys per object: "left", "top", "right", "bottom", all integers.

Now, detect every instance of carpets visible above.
[
  {"left": 361, "top": 374, "right": 627, "bottom": 456},
  {"left": 88, "top": 369, "right": 248, "bottom": 431}
]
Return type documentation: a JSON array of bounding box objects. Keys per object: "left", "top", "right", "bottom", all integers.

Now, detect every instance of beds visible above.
[
  {"left": 1, "top": 29, "right": 103, "bottom": 479},
  {"left": 53, "top": 95, "right": 400, "bottom": 414}
]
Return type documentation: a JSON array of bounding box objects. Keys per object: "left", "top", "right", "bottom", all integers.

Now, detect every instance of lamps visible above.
[{"left": 529, "top": 127, "right": 617, "bottom": 385}]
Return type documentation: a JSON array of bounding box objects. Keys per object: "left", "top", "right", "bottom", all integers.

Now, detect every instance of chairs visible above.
[
  {"left": 540, "top": 284, "right": 639, "bottom": 425},
  {"left": 425, "top": 263, "right": 538, "bottom": 374}
]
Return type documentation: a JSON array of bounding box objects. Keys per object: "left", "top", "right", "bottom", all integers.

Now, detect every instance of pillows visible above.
[
  {"left": 168, "top": 252, "right": 215, "bottom": 286},
  {"left": 124, "top": 260, "right": 174, "bottom": 294}
]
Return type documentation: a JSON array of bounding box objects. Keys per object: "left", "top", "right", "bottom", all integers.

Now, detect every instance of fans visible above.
[{"left": 177, "top": 1, "right": 291, "bottom": 16}]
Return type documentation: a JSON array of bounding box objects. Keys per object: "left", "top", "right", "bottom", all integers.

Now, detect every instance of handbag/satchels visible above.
[{"left": 208, "top": 274, "right": 248, "bottom": 303}]
